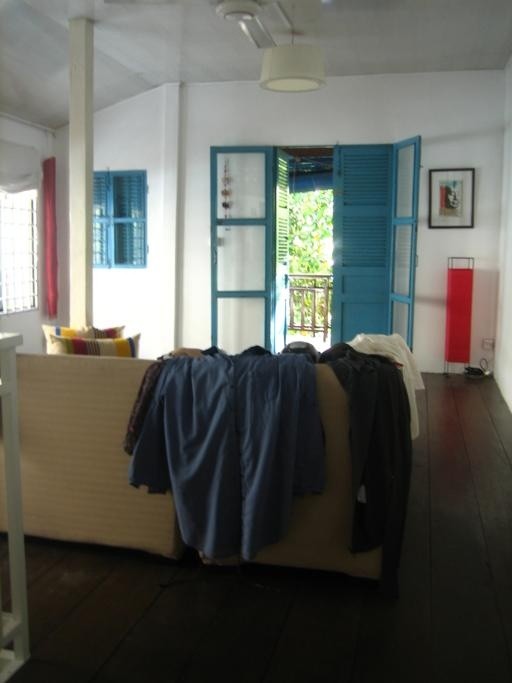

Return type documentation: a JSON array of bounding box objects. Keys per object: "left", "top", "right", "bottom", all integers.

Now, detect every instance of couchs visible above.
[{"left": 3, "top": 338, "right": 407, "bottom": 585}]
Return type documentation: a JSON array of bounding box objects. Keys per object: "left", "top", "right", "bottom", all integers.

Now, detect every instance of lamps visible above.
[{"left": 256, "top": 28, "right": 326, "bottom": 94}]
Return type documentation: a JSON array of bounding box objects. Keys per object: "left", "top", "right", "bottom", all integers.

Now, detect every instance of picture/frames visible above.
[{"left": 428, "top": 167, "right": 475, "bottom": 228}]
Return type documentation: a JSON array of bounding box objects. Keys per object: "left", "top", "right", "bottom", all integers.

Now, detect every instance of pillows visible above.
[{"left": 40, "top": 324, "right": 143, "bottom": 361}]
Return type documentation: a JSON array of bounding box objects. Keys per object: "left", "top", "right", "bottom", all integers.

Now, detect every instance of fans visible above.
[{"left": 208, "top": 0, "right": 285, "bottom": 52}]
